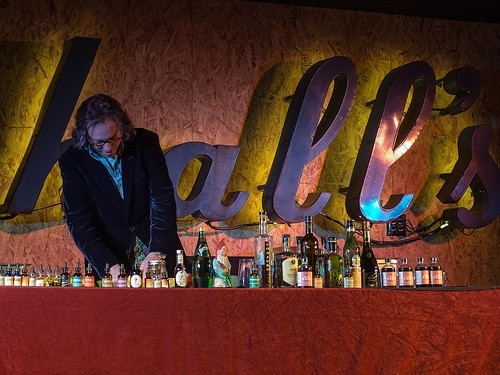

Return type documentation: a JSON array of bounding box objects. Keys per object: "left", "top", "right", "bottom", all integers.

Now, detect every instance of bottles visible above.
[
  {"left": 130, "top": 263, "right": 143, "bottom": 288},
  {"left": 430, "top": 257, "right": 443, "bottom": 287},
  {"left": 192, "top": 227, "right": 214, "bottom": 288},
  {"left": 52, "top": 265, "right": 60, "bottom": 287},
  {"left": 83, "top": 263, "right": 95, "bottom": 287},
  {"left": 415, "top": 258, "right": 430, "bottom": 288},
  {"left": 174, "top": 250, "right": 187, "bottom": 288},
  {"left": 72, "top": 263, "right": 83, "bottom": 287},
  {"left": 44, "top": 265, "right": 53, "bottom": 287},
  {"left": 296, "top": 215, "right": 324, "bottom": 289},
  {"left": 361, "top": 221, "right": 381, "bottom": 288},
  {"left": 275, "top": 234, "right": 299, "bottom": 288},
  {"left": 101, "top": 263, "right": 113, "bottom": 288},
  {"left": 35, "top": 265, "right": 43, "bottom": 286},
  {"left": 117, "top": 264, "right": 127, "bottom": 288},
  {"left": 145, "top": 260, "right": 169, "bottom": 288},
  {"left": 325, "top": 237, "right": 342, "bottom": 288},
  {"left": 249, "top": 269, "right": 260, "bottom": 289},
  {"left": 60, "top": 262, "right": 70, "bottom": 287},
  {"left": 254, "top": 212, "right": 274, "bottom": 289},
  {"left": 398, "top": 258, "right": 415, "bottom": 287},
  {"left": 0, "top": 263, "right": 35, "bottom": 286},
  {"left": 343, "top": 220, "right": 361, "bottom": 288},
  {"left": 382, "top": 258, "right": 398, "bottom": 288}
]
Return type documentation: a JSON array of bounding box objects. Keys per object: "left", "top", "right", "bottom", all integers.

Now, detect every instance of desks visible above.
[{"left": 0, "top": 285, "right": 500, "bottom": 375}]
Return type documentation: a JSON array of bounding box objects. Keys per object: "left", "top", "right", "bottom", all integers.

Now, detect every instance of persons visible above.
[{"left": 59, "top": 94, "right": 190, "bottom": 279}]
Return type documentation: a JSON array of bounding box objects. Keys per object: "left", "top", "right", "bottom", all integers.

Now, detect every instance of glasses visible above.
[{"left": 88, "top": 133, "right": 126, "bottom": 146}]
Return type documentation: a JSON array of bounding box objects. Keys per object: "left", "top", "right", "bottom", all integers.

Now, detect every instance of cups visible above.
[{"left": 237, "top": 259, "right": 254, "bottom": 288}]
